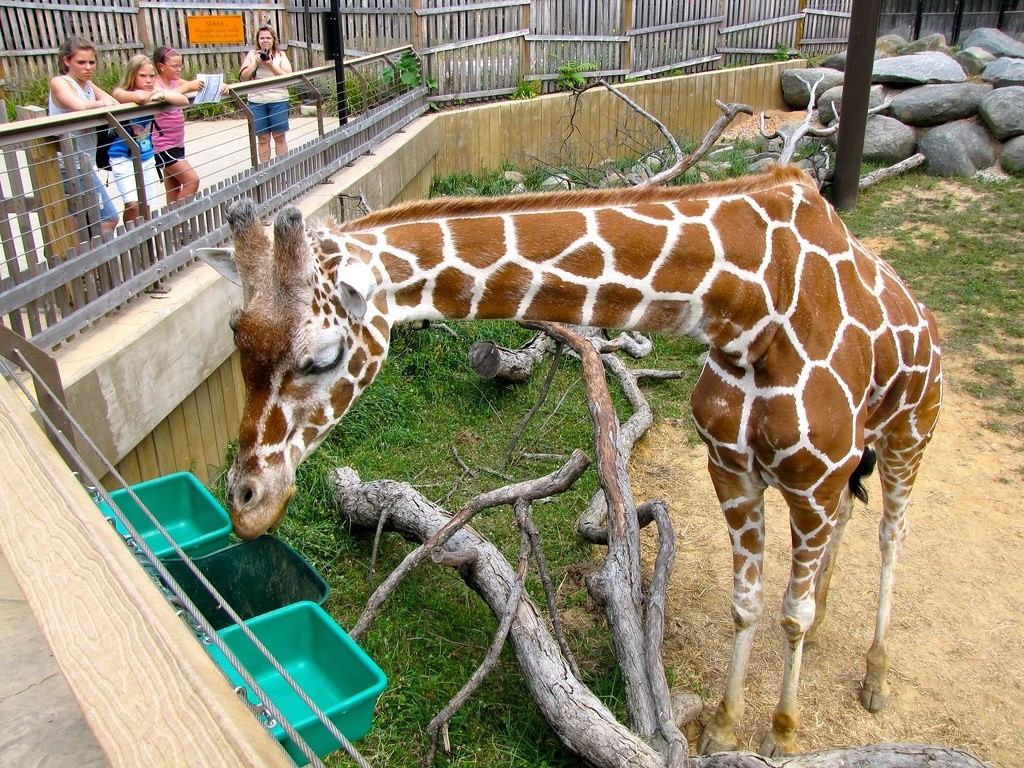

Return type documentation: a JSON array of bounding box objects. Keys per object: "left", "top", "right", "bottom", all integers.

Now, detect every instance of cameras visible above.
[{"left": 260, "top": 48, "right": 273, "bottom": 61}]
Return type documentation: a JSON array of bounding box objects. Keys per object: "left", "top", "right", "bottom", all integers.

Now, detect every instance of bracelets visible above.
[{"left": 162, "top": 89, "right": 166, "bottom": 101}]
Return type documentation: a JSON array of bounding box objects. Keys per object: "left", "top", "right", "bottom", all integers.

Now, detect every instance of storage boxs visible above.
[
  {"left": 94, "top": 470, "right": 233, "bottom": 560},
  {"left": 159, "top": 534, "right": 330, "bottom": 636},
  {"left": 198, "top": 600, "right": 388, "bottom": 768}
]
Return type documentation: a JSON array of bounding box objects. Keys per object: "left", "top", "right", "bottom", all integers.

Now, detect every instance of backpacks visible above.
[{"left": 96, "top": 115, "right": 164, "bottom": 171}]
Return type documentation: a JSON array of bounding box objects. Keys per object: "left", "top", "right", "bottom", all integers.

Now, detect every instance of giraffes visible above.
[{"left": 191, "top": 160, "right": 944, "bottom": 763}]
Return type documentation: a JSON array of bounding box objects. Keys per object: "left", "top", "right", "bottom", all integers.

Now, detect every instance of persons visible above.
[
  {"left": 48, "top": 36, "right": 121, "bottom": 287},
  {"left": 151, "top": 46, "right": 229, "bottom": 244},
  {"left": 106, "top": 54, "right": 189, "bottom": 265},
  {"left": 238, "top": 25, "right": 293, "bottom": 183}
]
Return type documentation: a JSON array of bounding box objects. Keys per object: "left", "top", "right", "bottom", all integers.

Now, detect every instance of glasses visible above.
[{"left": 168, "top": 64, "right": 186, "bottom": 69}]
